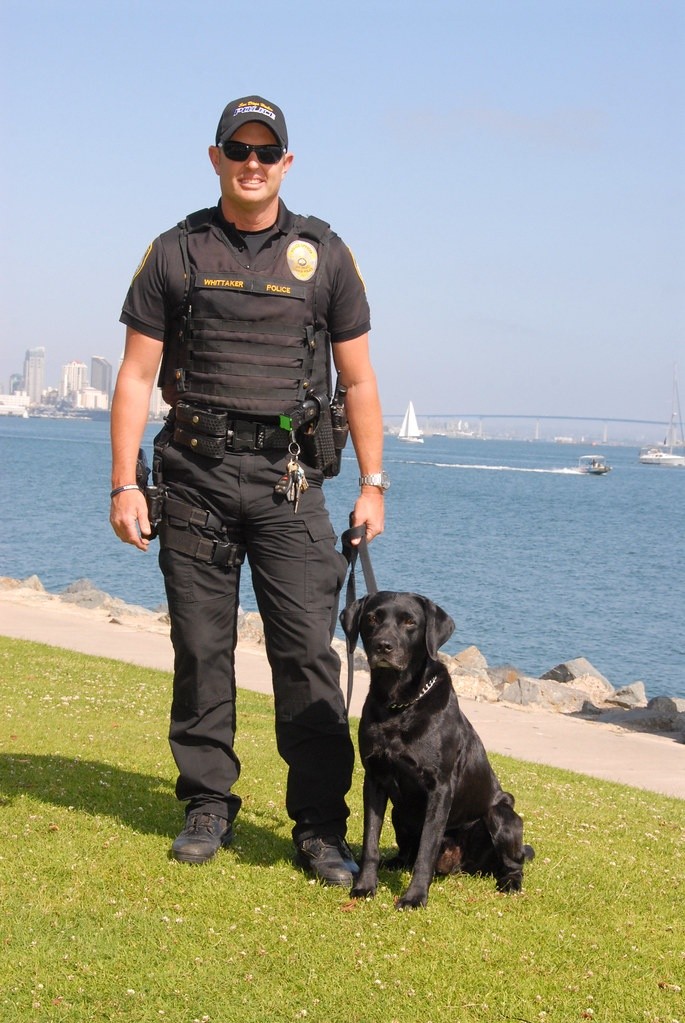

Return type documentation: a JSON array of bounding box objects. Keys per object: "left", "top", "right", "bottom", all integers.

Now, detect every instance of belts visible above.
[{"left": 226, "top": 419, "right": 301, "bottom": 455}]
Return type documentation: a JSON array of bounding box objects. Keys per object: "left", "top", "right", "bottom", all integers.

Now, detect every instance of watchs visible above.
[{"left": 358, "top": 470, "right": 391, "bottom": 492}]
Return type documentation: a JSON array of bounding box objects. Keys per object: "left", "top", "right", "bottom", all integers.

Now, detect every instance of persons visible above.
[{"left": 109, "top": 95, "right": 392, "bottom": 895}]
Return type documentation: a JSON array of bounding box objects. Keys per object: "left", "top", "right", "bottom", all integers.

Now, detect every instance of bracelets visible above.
[{"left": 110, "top": 484, "right": 139, "bottom": 497}]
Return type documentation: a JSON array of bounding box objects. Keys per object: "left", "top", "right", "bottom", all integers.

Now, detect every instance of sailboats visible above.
[
  {"left": 398, "top": 401, "right": 424, "bottom": 443},
  {"left": 639, "top": 363, "right": 684, "bottom": 466}
]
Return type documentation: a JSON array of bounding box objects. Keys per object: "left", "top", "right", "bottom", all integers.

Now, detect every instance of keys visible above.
[{"left": 273, "top": 456, "right": 309, "bottom": 513}]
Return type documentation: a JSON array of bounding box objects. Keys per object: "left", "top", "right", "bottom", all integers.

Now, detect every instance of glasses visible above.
[{"left": 221, "top": 140, "right": 282, "bottom": 164}]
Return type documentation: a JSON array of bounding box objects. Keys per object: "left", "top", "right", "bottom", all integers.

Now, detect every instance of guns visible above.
[{"left": 280, "top": 391, "right": 338, "bottom": 480}]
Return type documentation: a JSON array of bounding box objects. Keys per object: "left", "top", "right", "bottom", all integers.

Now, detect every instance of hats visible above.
[{"left": 216, "top": 96, "right": 289, "bottom": 151}]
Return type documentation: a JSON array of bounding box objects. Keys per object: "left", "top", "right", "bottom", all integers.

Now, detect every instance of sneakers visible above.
[
  {"left": 170, "top": 812, "right": 233, "bottom": 862},
  {"left": 301, "top": 832, "right": 360, "bottom": 886}
]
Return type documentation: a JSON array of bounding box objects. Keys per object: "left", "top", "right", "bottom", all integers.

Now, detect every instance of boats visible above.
[{"left": 578, "top": 455, "right": 612, "bottom": 474}]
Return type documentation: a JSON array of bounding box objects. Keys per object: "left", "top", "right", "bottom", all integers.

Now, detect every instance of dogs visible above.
[{"left": 338, "top": 590, "right": 536, "bottom": 911}]
